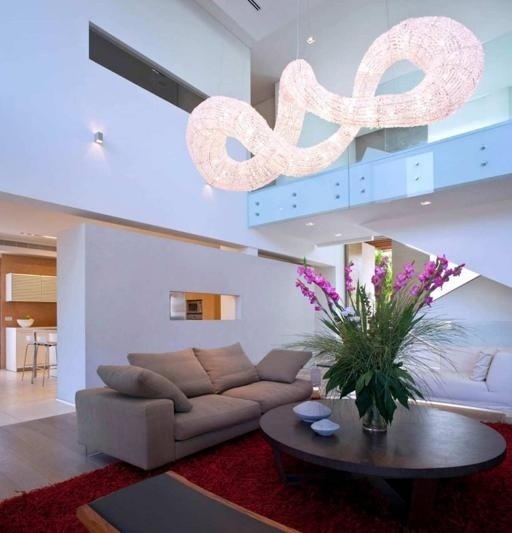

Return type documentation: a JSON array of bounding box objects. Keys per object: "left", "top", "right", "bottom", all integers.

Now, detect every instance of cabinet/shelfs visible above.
[
  {"left": 5, "top": 273, "right": 56, "bottom": 302},
  {"left": 5, "top": 327, "right": 58, "bottom": 371}
]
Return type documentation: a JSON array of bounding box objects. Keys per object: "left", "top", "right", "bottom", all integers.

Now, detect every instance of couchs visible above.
[
  {"left": 75, "top": 380, "right": 313, "bottom": 470},
  {"left": 400, "top": 347, "right": 512, "bottom": 425}
]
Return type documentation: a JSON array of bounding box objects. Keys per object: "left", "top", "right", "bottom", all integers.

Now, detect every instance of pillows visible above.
[
  {"left": 97, "top": 364, "right": 191, "bottom": 413},
  {"left": 470, "top": 349, "right": 494, "bottom": 381},
  {"left": 257, "top": 347, "right": 312, "bottom": 383},
  {"left": 127, "top": 349, "right": 216, "bottom": 397},
  {"left": 193, "top": 342, "right": 259, "bottom": 393}
]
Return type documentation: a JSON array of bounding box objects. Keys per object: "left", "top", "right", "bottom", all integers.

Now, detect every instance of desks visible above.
[
  {"left": 260, "top": 401, "right": 507, "bottom": 503},
  {"left": 32, "top": 329, "right": 57, "bottom": 378}
]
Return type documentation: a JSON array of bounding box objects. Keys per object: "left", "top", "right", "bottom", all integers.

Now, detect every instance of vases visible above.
[{"left": 361, "top": 398, "right": 389, "bottom": 434}]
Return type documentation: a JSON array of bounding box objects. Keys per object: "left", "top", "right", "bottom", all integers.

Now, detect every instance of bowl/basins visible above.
[
  {"left": 292, "top": 405, "right": 332, "bottom": 423},
  {"left": 17, "top": 318, "right": 35, "bottom": 328},
  {"left": 310, "top": 422, "right": 340, "bottom": 436}
]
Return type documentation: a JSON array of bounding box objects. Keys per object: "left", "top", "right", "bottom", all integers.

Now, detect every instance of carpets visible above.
[{"left": 0, "top": 423, "right": 511, "bottom": 533}]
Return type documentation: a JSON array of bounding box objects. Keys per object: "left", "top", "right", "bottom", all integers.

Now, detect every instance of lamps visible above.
[{"left": 95, "top": 130, "right": 103, "bottom": 143}]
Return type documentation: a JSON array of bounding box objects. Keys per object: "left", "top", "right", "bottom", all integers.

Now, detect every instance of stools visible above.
[
  {"left": 22, "top": 341, "right": 58, "bottom": 387},
  {"left": 78, "top": 469, "right": 303, "bottom": 533}
]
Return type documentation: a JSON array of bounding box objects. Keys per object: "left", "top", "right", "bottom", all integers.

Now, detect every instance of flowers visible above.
[{"left": 296, "top": 254, "right": 464, "bottom": 424}]
